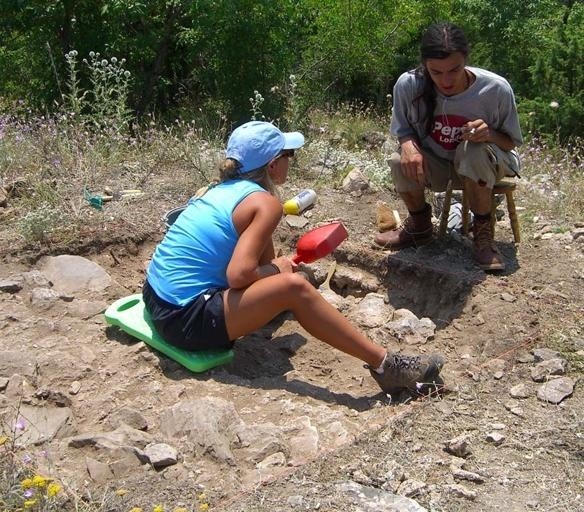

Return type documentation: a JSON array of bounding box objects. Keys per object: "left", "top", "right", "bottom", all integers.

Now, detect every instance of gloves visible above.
[{"left": 446, "top": 202, "right": 474, "bottom": 231}]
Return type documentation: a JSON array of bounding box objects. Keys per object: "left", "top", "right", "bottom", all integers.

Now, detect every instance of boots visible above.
[
  {"left": 473, "top": 215, "right": 506, "bottom": 271},
  {"left": 372, "top": 205, "right": 432, "bottom": 251}
]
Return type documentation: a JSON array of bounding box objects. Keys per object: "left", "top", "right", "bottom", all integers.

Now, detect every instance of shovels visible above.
[{"left": 293, "top": 223, "right": 349, "bottom": 264}]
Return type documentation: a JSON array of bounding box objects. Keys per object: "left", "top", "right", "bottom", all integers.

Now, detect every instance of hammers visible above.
[{"left": 104, "top": 186, "right": 141, "bottom": 195}]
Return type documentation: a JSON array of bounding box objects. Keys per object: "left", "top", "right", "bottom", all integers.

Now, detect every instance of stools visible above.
[{"left": 438, "top": 177, "right": 521, "bottom": 248}]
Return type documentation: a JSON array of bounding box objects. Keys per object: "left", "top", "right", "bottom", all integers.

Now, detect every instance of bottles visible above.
[{"left": 284, "top": 188, "right": 318, "bottom": 214}]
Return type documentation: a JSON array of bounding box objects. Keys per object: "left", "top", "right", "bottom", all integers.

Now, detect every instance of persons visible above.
[
  {"left": 369, "top": 21, "right": 526, "bottom": 274},
  {"left": 139, "top": 119, "right": 447, "bottom": 403}
]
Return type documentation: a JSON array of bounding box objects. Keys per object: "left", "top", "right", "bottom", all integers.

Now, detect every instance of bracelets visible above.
[{"left": 268, "top": 261, "right": 282, "bottom": 274}]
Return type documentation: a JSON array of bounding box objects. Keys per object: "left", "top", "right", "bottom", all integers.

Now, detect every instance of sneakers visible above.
[{"left": 369, "top": 347, "right": 444, "bottom": 394}]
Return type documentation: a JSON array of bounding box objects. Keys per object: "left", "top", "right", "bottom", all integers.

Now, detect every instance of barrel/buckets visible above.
[{"left": 163, "top": 206, "right": 186, "bottom": 238}]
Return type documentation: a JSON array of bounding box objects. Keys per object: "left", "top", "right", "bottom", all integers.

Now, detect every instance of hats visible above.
[{"left": 226, "top": 121, "right": 305, "bottom": 173}]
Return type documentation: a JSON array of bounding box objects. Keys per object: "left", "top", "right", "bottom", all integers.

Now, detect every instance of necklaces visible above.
[{"left": 462, "top": 69, "right": 471, "bottom": 91}]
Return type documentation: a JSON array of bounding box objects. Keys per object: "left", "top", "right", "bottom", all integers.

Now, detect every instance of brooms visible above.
[{"left": 376, "top": 201, "right": 402, "bottom": 233}]
